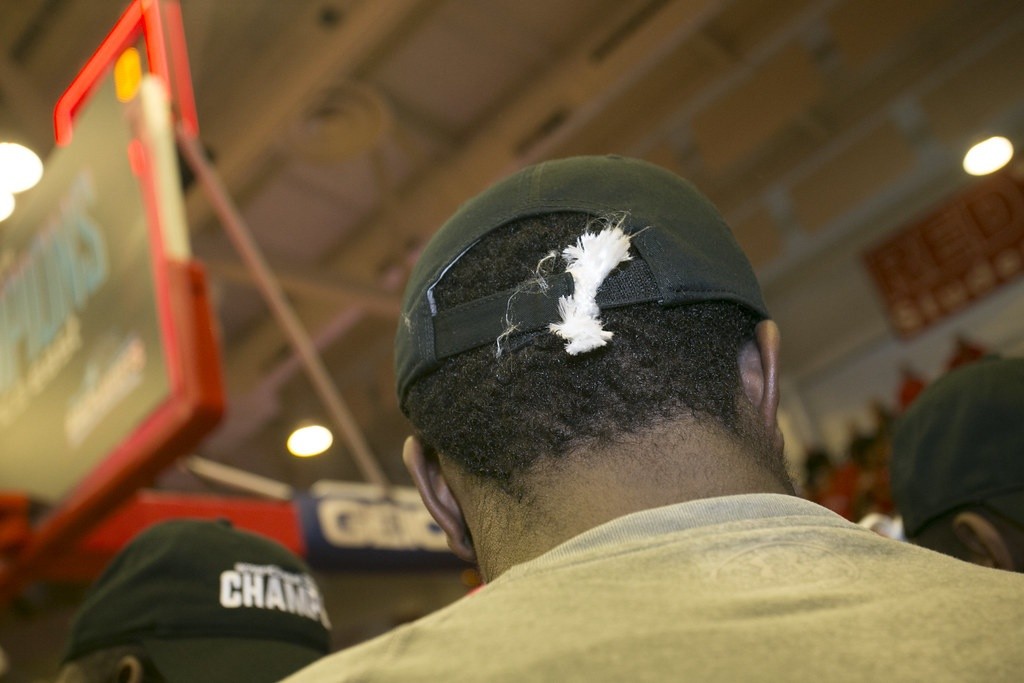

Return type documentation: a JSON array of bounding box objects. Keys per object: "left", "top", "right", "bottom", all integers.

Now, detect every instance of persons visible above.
[
  {"left": 888, "top": 356, "right": 1024, "bottom": 576},
  {"left": 53, "top": 518, "right": 334, "bottom": 683},
  {"left": 278, "top": 155, "right": 1024, "bottom": 683}
]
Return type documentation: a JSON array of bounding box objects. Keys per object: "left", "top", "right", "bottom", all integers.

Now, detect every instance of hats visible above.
[
  {"left": 891, "top": 356, "right": 1024, "bottom": 541},
  {"left": 392, "top": 153, "right": 772, "bottom": 414},
  {"left": 60, "top": 517, "right": 331, "bottom": 683}
]
